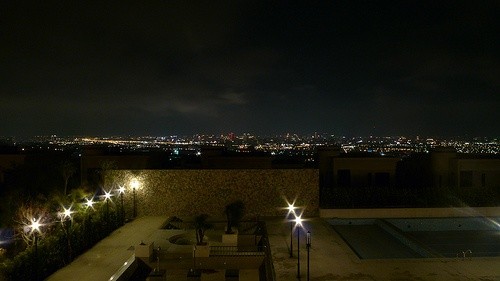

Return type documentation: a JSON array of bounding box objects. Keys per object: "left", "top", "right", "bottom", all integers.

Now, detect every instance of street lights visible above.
[
  {"left": 288, "top": 204, "right": 293, "bottom": 258},
  {"left": 306, "top": 230, "right": 312, "bottom": 281},
  {"left": 295, "top": 218, "right": 301, "bottom": 280}
]
